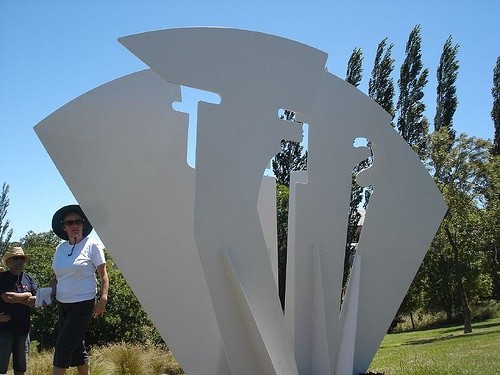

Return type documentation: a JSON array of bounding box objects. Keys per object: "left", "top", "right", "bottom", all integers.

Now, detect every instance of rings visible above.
[{"left": 94, "top": 312, "right": 101, "bottom": 317}]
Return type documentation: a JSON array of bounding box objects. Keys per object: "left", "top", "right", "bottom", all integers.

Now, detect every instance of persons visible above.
[
  {"left": 0, "top": 247, "right": 39, "bottom": 375},
  {"left": 0, "top": 312, "right": 12, "bottom": 322},
  {"left": 29, "top": 211, "right": 109, "bottom": 375}
]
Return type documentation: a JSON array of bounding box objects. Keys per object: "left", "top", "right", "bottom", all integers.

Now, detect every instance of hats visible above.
[
  {"left": 52, "top": 205, "right": 93, "bottom": 240},
  {"left": 3, "top": 247, "right": 30, "bottom": 269}
]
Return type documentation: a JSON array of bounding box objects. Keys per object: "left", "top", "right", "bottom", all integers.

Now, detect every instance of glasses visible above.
[{"left": 61, "top": 219, "right": 84, "bottom": 226}]
